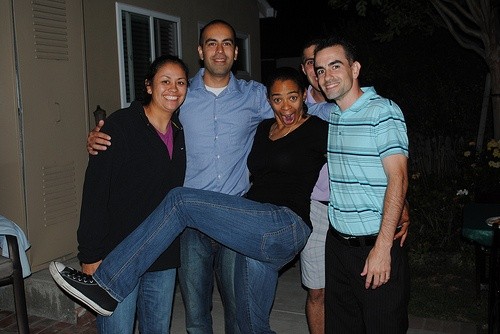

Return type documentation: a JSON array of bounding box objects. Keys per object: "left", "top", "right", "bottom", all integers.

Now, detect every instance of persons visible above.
[
  {"left": 300, "top": 40, "right": 330, "bottom": 334},
  {"left": 49, "top": 66, "right": 411, "bottom": 334},
  {"left": 77, "top": 55, "right": 191, "bottom": 334},
  {"left": 87, "top": 19, "right": 339, "bottom": 334},
  {"left": 313, "top": 40, "right": 410, "bottom": 334}
]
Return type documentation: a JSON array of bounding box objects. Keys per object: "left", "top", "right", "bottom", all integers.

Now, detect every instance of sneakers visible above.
[{"left": 49, "top": 262, "right": 117, "bottom": 316}]
